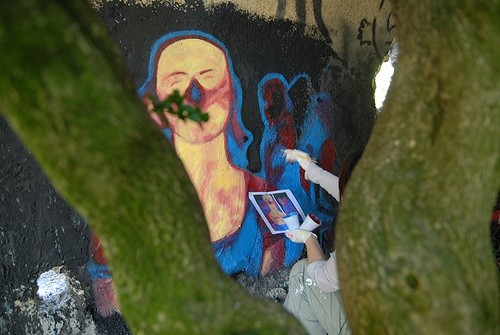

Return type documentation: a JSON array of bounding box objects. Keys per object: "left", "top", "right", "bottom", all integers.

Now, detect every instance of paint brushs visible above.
[{"left": 281, "top": 148, "right": 318, "bottom": 163}]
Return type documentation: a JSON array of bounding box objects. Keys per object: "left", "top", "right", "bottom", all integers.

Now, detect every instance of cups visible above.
[
  {"left": 300, "top": 213, "right": 322, "bottom": 230},
  {"left": 283, "top": 212, "right": 300, "bottom": 230}
]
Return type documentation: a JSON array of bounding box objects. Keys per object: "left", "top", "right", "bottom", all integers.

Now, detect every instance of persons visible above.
[{"left": 282, "top": 149, "right": 369, "bottom": 335}]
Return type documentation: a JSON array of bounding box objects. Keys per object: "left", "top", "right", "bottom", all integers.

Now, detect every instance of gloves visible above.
[
  {"left": 285, "top": 229, "right": 318, "bottom": 244},
  {"left": 284, "top": 148, "right": 310, "bottom": 170}
]
